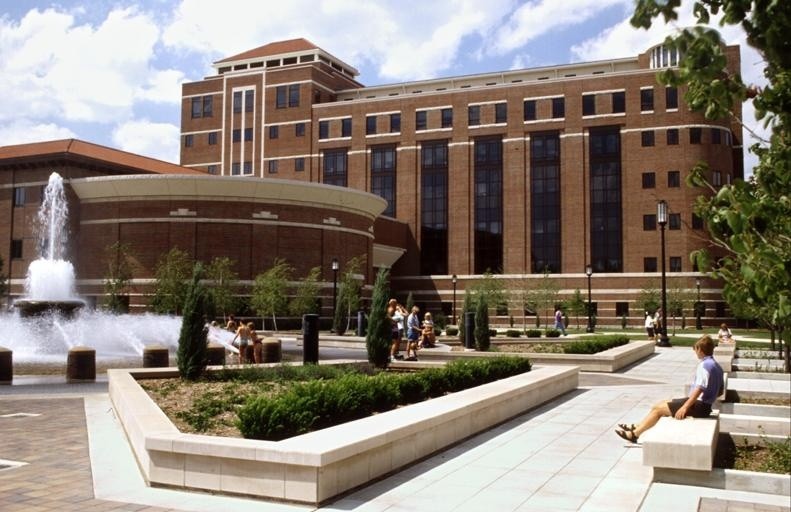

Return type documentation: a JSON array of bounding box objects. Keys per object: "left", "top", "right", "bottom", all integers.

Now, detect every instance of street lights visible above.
[
  {"left": 696, "top": 277, "right": 703, "bottom": 329},
  {"left": 452, "top": 273, "right": 456, "bottom": 325},
  {"left": 585, "top": 264, "right": 593, "bottom": 332},
  {"left": 331, "top": 257, "right": 339, "bottom": 332},
  {"left": 656, "top": 199, "right": 673, "bottom": 346}
]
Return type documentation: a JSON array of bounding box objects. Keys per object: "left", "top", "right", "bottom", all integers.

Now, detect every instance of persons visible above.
[
  {"left": 645, "top": 310, "right": 655, "bottom": 341},
  {"left": 719, "top": 323, "right": 735, "bottom": 343},
  {"left": 388, "top": 298, "right": 437, "bottom": 362},
  {"left": 224, "top": 314, "right": 237, "bottom": 333},
  {"left": 554, "top": 305, "right": 569, "bottom": 336},
  {"left": 613, "top": 333, "right": 725, "bottom": 443},
  {"left": 247, "top": 322, "right": 264, "bottom": 365},
  {"left": 231, "top": 319, "right": 250, "bottom": 365},
  {"left": 654, "top": 307, "right": 664, "bottom": 338}
]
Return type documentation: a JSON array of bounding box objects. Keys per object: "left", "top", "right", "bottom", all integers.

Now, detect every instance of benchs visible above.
[
  {"left": 713, "top": 338, "right": 737, "bottom": 401},
  {"left": 642, "top": 402, "right": 721, "bottom": 485}
]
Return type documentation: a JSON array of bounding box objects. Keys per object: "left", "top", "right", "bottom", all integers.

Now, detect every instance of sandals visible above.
[
  {"left": 619, "top": 423, "right": 636, "bottom": 431},
  {"left": 614, "top": 429, "right": 638, "bottom": 443}
]
